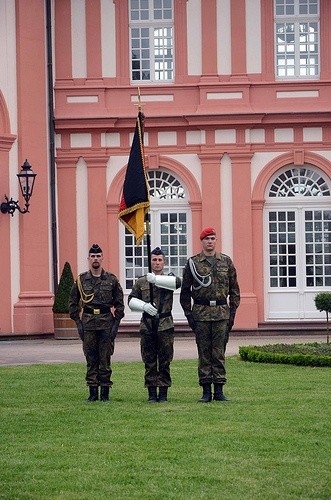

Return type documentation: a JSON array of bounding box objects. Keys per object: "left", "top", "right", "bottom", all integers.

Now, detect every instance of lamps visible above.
[{"left": 0, "top": 159, "right": 37, "bottom": 216}]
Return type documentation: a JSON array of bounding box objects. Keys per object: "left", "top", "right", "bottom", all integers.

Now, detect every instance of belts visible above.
[
  {"left": 83, "top": 308, "right": 111, "bottom": 315},
  {"left": 142, "top": 312, "right": 171, "bottom": 319},
  {"left": 194, "top": 299, "right": 227, "bottom": 307}
]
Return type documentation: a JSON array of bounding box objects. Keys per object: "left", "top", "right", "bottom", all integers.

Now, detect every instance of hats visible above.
[
  {"left": 200, "top": 227, "right": 216, "bottom": 241},
  {"left": 151, "top": 246, "right": 165, "bottom": 256},
  {"left": 89, "top": 243, "right": 102, "bottom": 254}
]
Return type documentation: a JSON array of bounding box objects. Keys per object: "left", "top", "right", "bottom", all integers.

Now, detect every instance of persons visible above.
[
  {"left": 70, "top": 244, "right": 125, "bottom": 402},
  {"left": 128, "top": 247, "right": 182, "bottom": 403},
  {"left": 180, "top": 228, "right": 241, "bottom": 402}
]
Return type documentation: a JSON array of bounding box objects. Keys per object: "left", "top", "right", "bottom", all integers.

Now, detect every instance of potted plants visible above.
[{"left": 52, "top": 262, "right": 80, "bottom": 340}]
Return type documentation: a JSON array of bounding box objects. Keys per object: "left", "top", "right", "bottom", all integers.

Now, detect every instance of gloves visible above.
[
  {"left": 185, "top": 314, "right": 197, "bottom": 331},
  {"left": 129, "top": 297, "right": 158, "bottom": 316},
  {"left": 146, "top": 272, "right": 176, "bottom": 291},
  {"left": 77, "top": 322, "right": 84, "bottom": 338},
  {"left": 227, "top": 315, "right": 235, "bottom": 330},
  {"left": 111, "top": 325, "right": 118, "bottom": 340}
]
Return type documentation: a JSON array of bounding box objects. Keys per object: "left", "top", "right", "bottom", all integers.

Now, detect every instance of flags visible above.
[{"left": 117, "top": 110, "right": 150, "bottom": 245}]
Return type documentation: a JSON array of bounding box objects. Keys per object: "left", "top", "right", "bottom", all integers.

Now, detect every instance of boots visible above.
[
  {"left": 100, "top": 386, "right": 109, "bottom": 402},
  {"left": 157, "top": 386, "right": 168, "bottom": 403},
  {"left": 213, "top": 383, "right": 230, "bottom": 401},
  {"left": 198, "top": 384, "right": 212, "bottom": 402},
  {"left": 146, "top": 386, "right": 157, "bottom": 403},
  {"left": 86, "top": 386, "right": 99, "bottom": 402}
]
